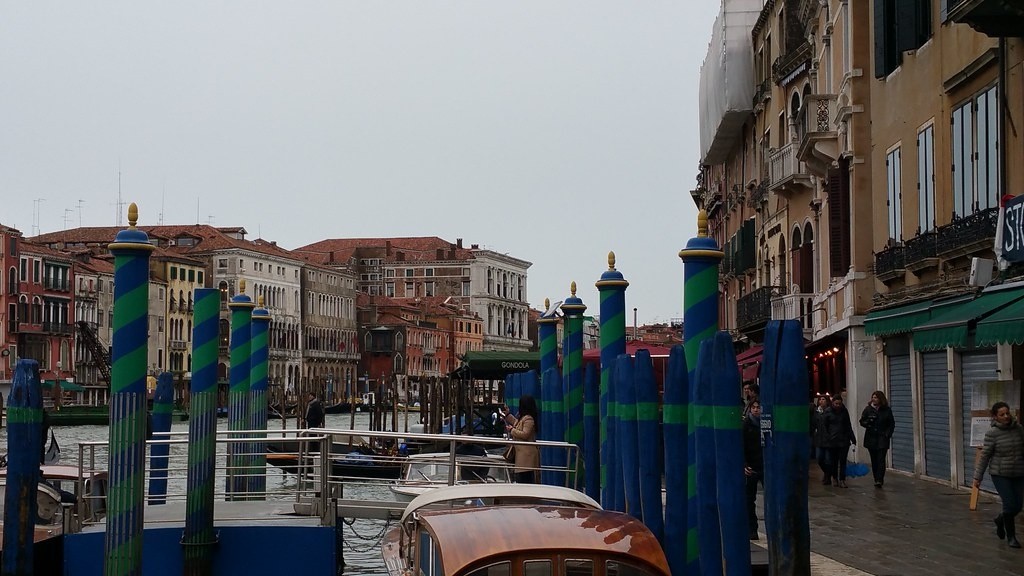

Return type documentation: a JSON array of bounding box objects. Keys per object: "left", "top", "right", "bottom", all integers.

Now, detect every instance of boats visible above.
[
  {"left": 263, "top": 428, "right": 490, "bottom": 478},
  {"left": 380, "top": 483, "right": 672, "bottom": 576},
  {"left": 396, "top": 403, "right": 445, "bottom": 412},
  {"left": 0, "top": 460, "right": 109, "bottom": 575},
  {"left": 388, "top": 451, "right": 513, "bottom": 504}
]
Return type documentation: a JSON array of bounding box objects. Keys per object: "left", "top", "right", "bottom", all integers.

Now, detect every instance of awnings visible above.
[{"left": 736, "top": 343, "right": 764, "bottom": 365}]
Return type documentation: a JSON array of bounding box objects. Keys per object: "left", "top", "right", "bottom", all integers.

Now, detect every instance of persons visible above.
[
  {"left": 453, "top": 431, "right": 490, "bottom": 482},
  {"left": 736, "top": 378, "right": 857, "bottom": 485},
  {"left": 502, "top": 396, "right": 540, "bottom": 483},
  {"left": 974, "top": 401, "right": 1024, "bottom": 548},
  {"left": 742, "top": 397, "right": 764, "bottom": 540},
  {"left": 304, "top": 391, "right": 323, "bottom": 452},
  {"left": 860, "top": 390, "right": 896, "bottom": 488}
]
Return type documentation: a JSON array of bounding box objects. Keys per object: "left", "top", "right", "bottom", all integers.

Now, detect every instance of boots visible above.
[
  {"left": 1003, "top": 512, "right": 1021, "bottom": 548},
  {"left": 994, "top": 511, "right": 1006, "bottom": 540}
]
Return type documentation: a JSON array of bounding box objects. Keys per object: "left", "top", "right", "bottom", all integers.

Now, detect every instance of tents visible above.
[
  {"left": 864, "top": 285, "right": 1024, "bottom": 354},
  {"left": 558, "top": 339, "right": 679, "bottom": 417},
  {"left": 461, "top": 349, "right": 542, "bottom": 409}
]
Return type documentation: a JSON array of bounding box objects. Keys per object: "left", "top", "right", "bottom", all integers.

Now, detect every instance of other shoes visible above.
[
  {"left": 833, "top": 478, "right": 838, "bottom": 487},
  {"left": 840, "top": 480, "right": 848, "bottom": 488},
  {"left": 874, "top": 482, "right": 882, "bottom": 488},
  {"left": 750, "top": 530, "right": 759, "bottom": 540}
]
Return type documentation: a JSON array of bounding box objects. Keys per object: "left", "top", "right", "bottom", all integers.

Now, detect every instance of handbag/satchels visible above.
[{"left": 503, "top": 445, "right": 515, "bottom": 462}]
